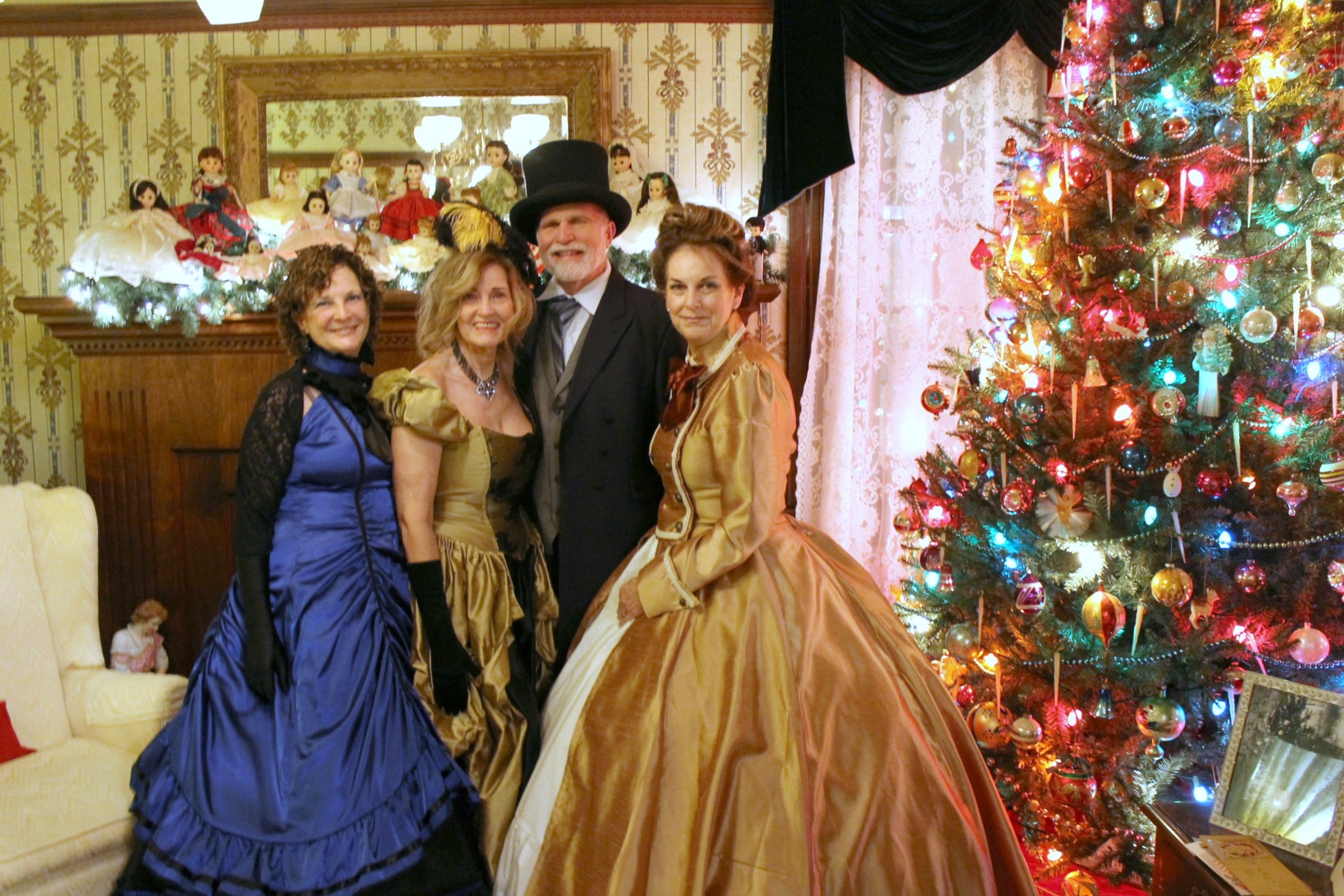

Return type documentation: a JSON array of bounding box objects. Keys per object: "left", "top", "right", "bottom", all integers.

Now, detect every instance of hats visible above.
[{"left": 509, "top": 139, "right": 634, "bottom": 248}]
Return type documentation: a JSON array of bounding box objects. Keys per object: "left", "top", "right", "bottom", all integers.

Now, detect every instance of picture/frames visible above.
[{"left": 1210, "top": 672, "right": 1344, "bottom": 869}]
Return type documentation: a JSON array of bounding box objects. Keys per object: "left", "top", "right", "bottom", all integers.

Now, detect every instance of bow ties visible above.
[{"left": 658, "top": 357, "right": 708, "bottom": 433}]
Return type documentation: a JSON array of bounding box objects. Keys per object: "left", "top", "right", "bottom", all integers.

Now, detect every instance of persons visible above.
[
  {"left": 367, "top": 207, "right": 561, "bottom": 885},
  {"left": 59, "top": 133, "right": 786, "bottom": 321},
  {"left": 109, "top": 598, "right": 171, "bottom": 676},
  {"left": 113, "top": 241, "right": 495, "bottom": 896},
  {"left": 492, "top": 198, "right": 1042, "bottom": 896},
  {"left": 501, "top": 138, "right": 691, "bottom": 690}
]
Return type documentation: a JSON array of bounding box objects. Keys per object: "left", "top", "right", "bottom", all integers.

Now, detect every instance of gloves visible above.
[
  {"left": 234, "top": 551, "right": 291, "bottom": 709},
  {"left": 408, "top": 558, "right": 480, "bottom": 717}
]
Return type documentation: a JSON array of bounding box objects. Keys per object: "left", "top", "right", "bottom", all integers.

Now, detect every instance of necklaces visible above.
[{"left": 450, "top": 337, "right": 501, "bottom": 403}]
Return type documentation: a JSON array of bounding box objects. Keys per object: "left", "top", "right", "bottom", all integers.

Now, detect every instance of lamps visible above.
[{"left": 412, "top": 96, "right": 570, "bottom": 204}]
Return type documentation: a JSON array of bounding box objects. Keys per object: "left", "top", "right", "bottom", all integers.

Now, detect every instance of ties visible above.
[{"left": 547, "top": 297, "right": 580, "bottom": 384}]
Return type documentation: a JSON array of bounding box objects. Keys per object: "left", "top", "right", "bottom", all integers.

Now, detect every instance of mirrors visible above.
[{"left": 216, "top": 47, "right": 615, "bottom": 208}]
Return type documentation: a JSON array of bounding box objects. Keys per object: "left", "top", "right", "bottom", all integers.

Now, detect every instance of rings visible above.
[{"left": 620, "top": 607, "right": 626, "bottom": 618}]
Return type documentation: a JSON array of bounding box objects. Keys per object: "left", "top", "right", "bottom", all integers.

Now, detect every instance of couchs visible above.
[{"left": 1, "top": 480, "right": 193, "bottom": 896}]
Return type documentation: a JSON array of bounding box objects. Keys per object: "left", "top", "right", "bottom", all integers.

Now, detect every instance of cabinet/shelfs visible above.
[{"left": 1139, "top": 802, "right": 1344, "bottom": 896}]
